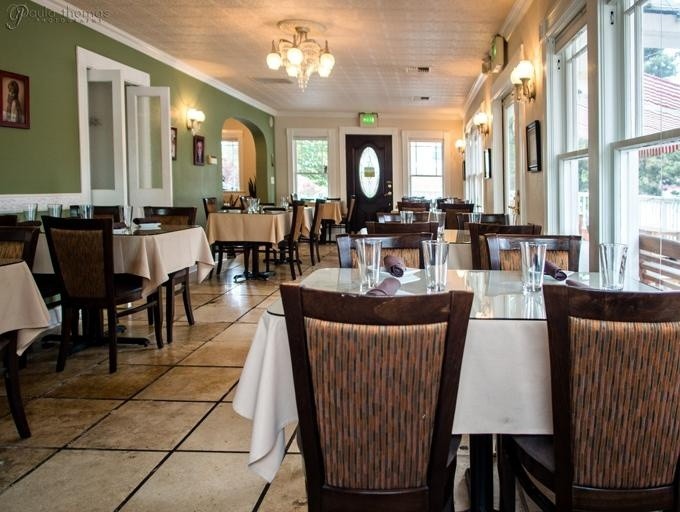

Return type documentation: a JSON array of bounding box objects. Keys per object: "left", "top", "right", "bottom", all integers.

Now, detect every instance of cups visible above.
[
  {"left": 431, "top": 210, "right": 447, "bottom": 234},
  {"left": 121, "top": 205, "right": 133, "bottom": 229},
  {"left": 597, "top": 241, "right": 626, "bottom": 293},
  {"left": 422, "top": 238, "right": 450, "bottom": 295},
  {"left": 78, "top": 200, "right": 93, "bottom": 219},
  {"left": 23, "top": 202, "right": 38, "bottom": 221},
  {"left": 46, "top": 203, "right": 63, "bottom": 218},
  {"left": 358, "top": 236, "right": 382, "bottom": 289},
  {"left": 519, "top": 238, "right": 545, "bottom": 293},
  {"left": 400, "top": 210, "right": 413, "bottom": 224},
  {"left": 468, "top": 211, "right": 482, "bottom": 224}
]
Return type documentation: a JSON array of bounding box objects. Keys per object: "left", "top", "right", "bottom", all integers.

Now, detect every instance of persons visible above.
[
  {"left": 194, "top": 141, "right": 204, "bottom": 163},
  {"left": 3, "top": 81, "right": 23, "bottom": 122}
]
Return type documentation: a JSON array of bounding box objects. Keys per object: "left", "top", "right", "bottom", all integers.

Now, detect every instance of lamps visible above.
[
  {"left": 474, "top": 113, "right": 489, "bottom": 135},
  {"left": 266, "top": 20, "right": 335, "bottom": 93},
  {"left": 510, "top": 60, "right": 536, "bottom": 102},
  {"left": 186, "top": 110, "right": 205, "bottom": 136},
  {"left": 456, "top": 139, "right": 464, "bottom": 154}
]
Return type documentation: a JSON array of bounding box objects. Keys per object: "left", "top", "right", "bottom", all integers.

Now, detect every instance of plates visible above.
[
  {"left": 138, "top": 222, "right": 162, "bottom": 229},
  {"left": 371, "top": 264, "right": 421, "bottom": 275}
]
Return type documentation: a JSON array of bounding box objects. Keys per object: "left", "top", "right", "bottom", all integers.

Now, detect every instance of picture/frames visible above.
[
  {"left": 0, "top": 69, "right": 31, "bottom": 129},
  {"left": 171, "top": 127, "right": 204, "bottom": 166},
  {"left": 526, "top": 120, "right": 541, "bottom": 172},
  {"left": 484, "top": 148, "right": 490, "bottom": 179}
]
